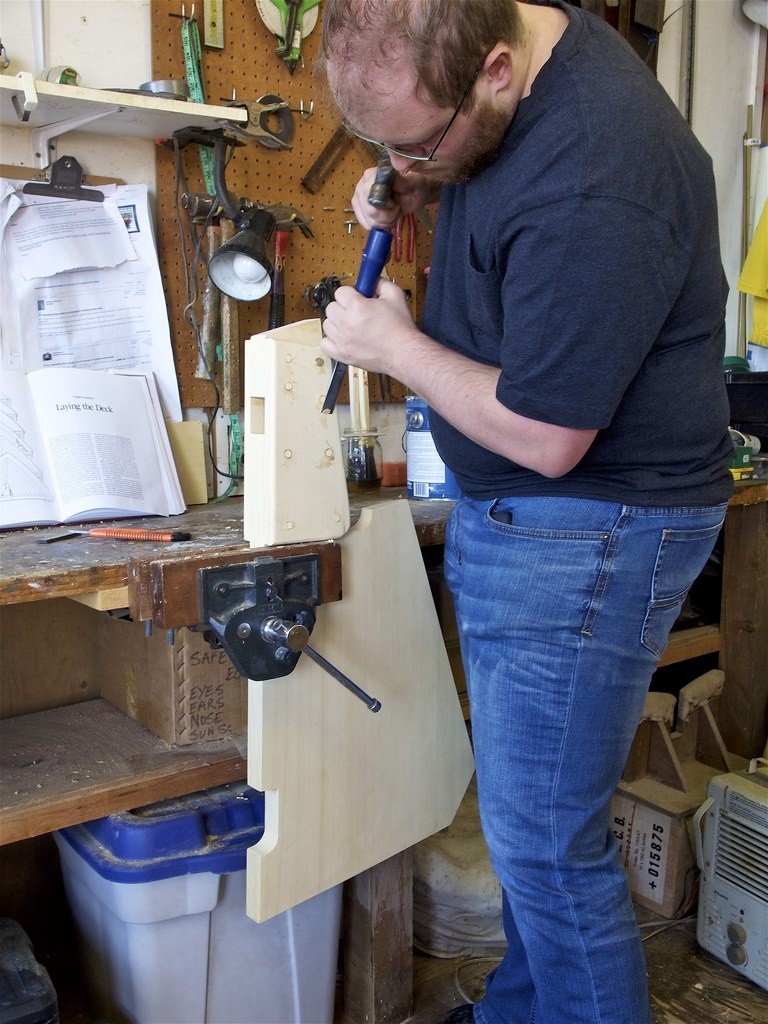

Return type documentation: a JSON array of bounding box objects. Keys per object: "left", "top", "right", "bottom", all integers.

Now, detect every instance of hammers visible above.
[
  {"left": 182, "top": 194, "right": 257, "bottom": 415},
  {"left": 254, "top": 204, "right": 315, "bottom": 330},
  {"left": 367, "top": 151, "right": 407, "bottom": 215}
]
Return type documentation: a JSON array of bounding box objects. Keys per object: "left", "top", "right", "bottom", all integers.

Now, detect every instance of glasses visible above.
[{"left": 338, "top": 44, "right": 499, "bottom": 167}]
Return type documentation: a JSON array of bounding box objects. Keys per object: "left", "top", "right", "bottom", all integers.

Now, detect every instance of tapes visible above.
[
  {"left": 139, "top": 80, "right": 188, "bottom": 98},
  {"left": 255, "top": 95, "right": 294, "bottom": 143}
]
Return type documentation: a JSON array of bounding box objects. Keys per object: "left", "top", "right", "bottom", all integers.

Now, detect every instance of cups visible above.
[{"left": 728, "top": 428, "right": 761, "bottom": 457}]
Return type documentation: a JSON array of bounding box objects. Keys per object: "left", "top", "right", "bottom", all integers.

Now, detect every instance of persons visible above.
[{"left": 320, "top": 0, "right": 734, "bottom": 1024}]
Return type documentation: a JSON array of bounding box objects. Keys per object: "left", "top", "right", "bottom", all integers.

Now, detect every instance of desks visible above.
[{"left": 0, "top": 468, "right": 768, "bottom": 1024}]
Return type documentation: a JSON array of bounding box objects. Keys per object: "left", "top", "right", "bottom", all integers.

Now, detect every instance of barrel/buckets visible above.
[{"left": 401, "top": 394, "right": 461, "bottom": 503}]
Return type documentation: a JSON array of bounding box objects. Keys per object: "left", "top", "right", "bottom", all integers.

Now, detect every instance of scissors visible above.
[{"left": 305, "top": 276, "right": 342, "bottom": 319}]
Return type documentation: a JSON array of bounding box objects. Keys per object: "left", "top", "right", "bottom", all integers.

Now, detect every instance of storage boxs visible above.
[{"left": 53, "top": 780, "right": 342, "bottom": 1024}]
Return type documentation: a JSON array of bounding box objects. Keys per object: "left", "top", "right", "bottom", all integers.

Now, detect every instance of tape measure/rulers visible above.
[{"left": 181, "top": 18, "right": 221, "bottom": 198}]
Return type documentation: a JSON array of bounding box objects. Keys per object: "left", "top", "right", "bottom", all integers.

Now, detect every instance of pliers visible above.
[{"left": 395, "top": 209, "right": 415, "bottom": 262}]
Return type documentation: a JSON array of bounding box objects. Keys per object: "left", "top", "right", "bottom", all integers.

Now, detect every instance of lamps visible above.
[{"left": 159, "top": 127, "right": 276, "bottom": 301}]
